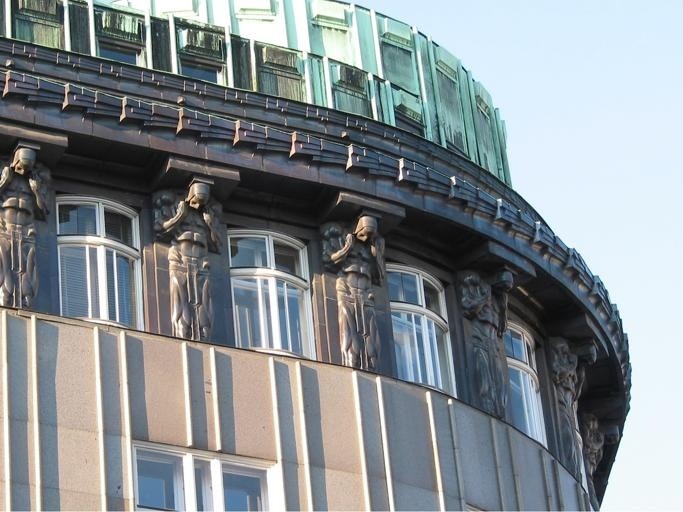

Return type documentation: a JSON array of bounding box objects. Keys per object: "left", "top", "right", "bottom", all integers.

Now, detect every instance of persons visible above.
[
  {"left": 585, "top": 424, "right": 619, "bottom": 512},
  {"left": 327, "top": 216, "right": 386, "bottom": 374},
  {"left": 0, "top": 147, "right": 50, "bottom": 310},
  {"left": 552, "top": 345, "right": 597, "bottom": 478},
  {"left": 161, "top": 182, "right": 223, "bottom": 343},
  {"left": 461, "top": 271, "right": 514, "bottom": 420}
]
